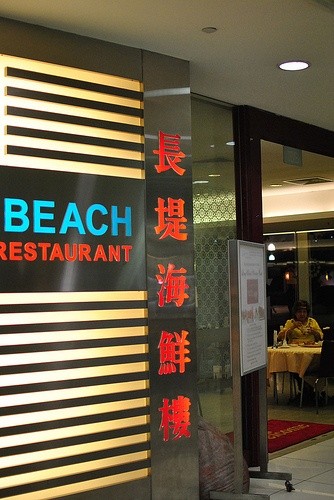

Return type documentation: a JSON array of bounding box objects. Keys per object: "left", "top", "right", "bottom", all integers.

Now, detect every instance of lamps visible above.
[
  {"left": 268, "top": 242, "right": 276, "bottom": 251},
  {"left": 268, "top": 252, "right": 276, "bottom": 260}
]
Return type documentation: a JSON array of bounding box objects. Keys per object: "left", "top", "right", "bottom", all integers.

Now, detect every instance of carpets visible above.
[{"left": 224, "top": 419, "right": 334, "bottom": 453}]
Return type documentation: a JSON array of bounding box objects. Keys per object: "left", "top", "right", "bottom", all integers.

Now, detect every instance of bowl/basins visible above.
[{"left": 301, "top": 326, "right": 310, "bottom": 332}]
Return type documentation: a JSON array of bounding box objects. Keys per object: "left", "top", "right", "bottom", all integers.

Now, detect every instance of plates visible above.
[{"left": 300, "top": 344, "right": 321, "bottom": 348}]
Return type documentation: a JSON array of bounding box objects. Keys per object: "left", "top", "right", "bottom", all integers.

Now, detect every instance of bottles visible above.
[{"left": 282, "top": 328, "right": 289, "bottom": 347}]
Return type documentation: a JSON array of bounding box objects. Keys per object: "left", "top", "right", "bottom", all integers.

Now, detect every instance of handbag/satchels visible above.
[{"left": 199, "top": 420, "right": 249, "bottom": 500}]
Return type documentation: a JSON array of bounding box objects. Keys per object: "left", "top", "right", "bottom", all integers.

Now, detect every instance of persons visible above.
[{"left": 277, "top": 300, "right": 323, "bottom": 404}]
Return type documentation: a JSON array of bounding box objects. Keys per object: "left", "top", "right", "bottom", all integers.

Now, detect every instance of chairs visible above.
[{"left": 299, "top": 326, "right": 334, "bottom": 415}]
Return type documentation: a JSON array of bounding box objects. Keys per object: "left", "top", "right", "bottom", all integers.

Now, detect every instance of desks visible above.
[{"left": 267, "top": 342, "right": 322, "bottom": 408}]
